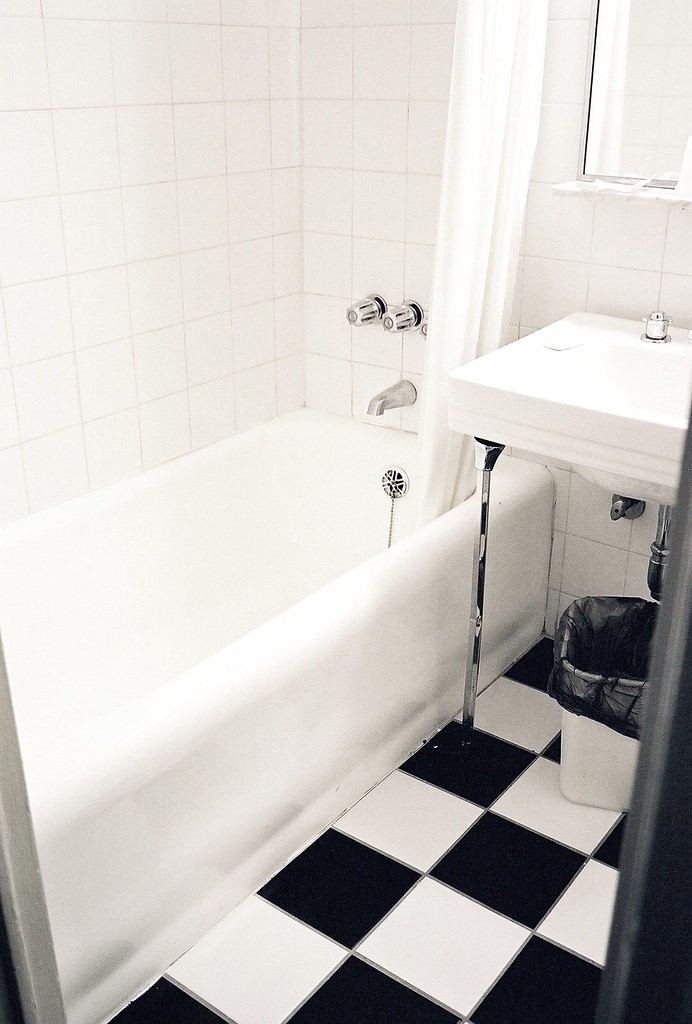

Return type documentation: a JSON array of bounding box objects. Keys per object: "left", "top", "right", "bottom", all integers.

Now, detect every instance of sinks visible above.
[{"left": 444, "top": 310, "right": 691, "bottom": 507}]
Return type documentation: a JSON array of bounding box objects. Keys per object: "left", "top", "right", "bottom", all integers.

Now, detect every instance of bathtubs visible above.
[{"left": 2, "top": 400, "right": 556, "bottom": 1020}]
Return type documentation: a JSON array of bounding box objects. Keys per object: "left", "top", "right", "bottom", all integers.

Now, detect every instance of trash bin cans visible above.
[{"left": 560, "top": 596, "right": 661, "bottom": 813}]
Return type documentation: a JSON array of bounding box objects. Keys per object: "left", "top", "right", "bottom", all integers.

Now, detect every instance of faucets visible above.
[{"left": 364, "top": 379, "right": 416, "bottom": 415}]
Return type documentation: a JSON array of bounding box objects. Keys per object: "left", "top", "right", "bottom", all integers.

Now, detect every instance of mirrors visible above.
[{"left": 577, "top": 0, "right": 692, "bottom": 189}]
